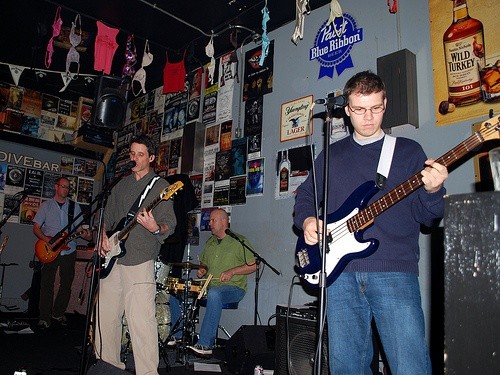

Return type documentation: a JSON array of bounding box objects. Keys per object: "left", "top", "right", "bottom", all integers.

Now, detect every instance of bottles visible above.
[{"left": 255, "top": 365, "right": 263, "bottom": 375}]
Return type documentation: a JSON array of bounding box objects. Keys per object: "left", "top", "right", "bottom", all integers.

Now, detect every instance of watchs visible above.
[{"left": 152, "top": 224, "right": 161, "bottom": 235}]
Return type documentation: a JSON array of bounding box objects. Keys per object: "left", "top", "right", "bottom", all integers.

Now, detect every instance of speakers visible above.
[
  {"left": 226, "top": 325, "right": 276, "bottom": 375},
  {"left": 94, "top": 74, "right": 130, "bottom": 130},
  {"left": 275, "top": 304, "right": 331, "bottom": 375}
]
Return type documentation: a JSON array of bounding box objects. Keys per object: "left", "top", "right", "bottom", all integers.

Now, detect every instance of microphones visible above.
[
  {"left": 224, "top": 229, "right": 238, "bottom": 240},
  {"left": 317, "top": 95, "right": 347, "bottom": 107},
  {"left": 21, "top": 187, "right": 36, "bottom": 197},
  {"left": 116, "top": 161, "right": 136, "bottom": 173}
]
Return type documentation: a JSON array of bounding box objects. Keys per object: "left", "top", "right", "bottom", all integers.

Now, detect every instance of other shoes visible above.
[
  {"left": 167, "top": 337, "right": 183, "bottom": 345},
  {"left": 53, "top": 315, "right": 68, "bottom": 323},
  {"left": 186, "top": 344, "right": 212, "bottom": 354},
  {"left": 39, "top": 319, "right": 50, "bottom": 329}
]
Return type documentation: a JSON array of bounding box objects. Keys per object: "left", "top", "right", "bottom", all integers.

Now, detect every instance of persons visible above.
[
  {"left": 293, "top": 71, "right": 448, "bottom": 375},
  {"left": 89, "top": 135, "right": 177, "bottom": 375},
  {"left": 33, "top": 178, "right": 93, "bottom": 330},
  {"left": 168, "top": 209, "right": 261, "bottom": 354}
]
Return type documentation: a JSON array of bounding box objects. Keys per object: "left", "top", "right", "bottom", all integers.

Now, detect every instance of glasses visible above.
[
  {"left": 57, "top": 184, "right": 70, "bottom": 190},
  {"left": 348, "top": 97, "right": 386, "bottom": 115}
]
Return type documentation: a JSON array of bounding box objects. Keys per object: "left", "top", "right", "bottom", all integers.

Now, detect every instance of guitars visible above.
[
  {"left": 293, "top": 113, "right": 500, "bottom": 289},
  {"left": 35, "top": 224, "right": 99, "bottom": 263},
  {"left": 95, "top": 181, "right": 184, "bottom": 280}
]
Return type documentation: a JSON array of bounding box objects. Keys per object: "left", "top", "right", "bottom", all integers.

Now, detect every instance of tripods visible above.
[{"left": 123, "top": 242, "right": 201, "bottom": 372}]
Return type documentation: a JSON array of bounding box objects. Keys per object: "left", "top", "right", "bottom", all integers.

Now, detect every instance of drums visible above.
[
  {"left": 151, "top": 260, "right": 171, "bottom": 283},
  {"left": 89, "top": 284, "right": 170, "bottom": 361},
  {"left": 166, "top": 276, "right": 208, "bottom": 295}
]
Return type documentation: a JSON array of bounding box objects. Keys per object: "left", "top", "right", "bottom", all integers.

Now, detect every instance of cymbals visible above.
[{"left": 168, "top": 262, "right": 203, "bottom": 269}]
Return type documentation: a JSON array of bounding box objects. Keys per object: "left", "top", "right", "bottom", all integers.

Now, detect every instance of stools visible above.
[{"left": 199, "top": 301, "right": 240, "bottom": 350}]
